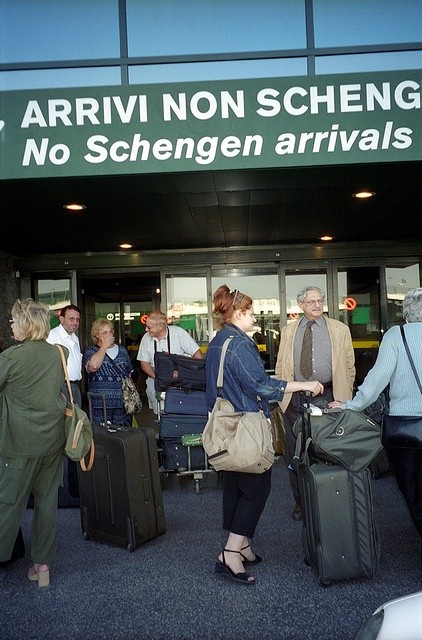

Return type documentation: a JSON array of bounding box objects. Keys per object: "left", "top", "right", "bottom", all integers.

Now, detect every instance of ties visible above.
[{"left": 299, "top": 321, "right": 315, "bottom": 380}]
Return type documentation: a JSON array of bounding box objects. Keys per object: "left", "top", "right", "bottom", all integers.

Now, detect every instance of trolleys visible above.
[{"left": 153, "top": 388, "right": 215, "bottom": 494}]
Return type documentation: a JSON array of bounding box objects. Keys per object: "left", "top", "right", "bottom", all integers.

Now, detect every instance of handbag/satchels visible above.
[
  {"left": 380, "top": 415, "right": 422, "bottom": 449},
  {"left": 121, "top": 374, "right": 142, "bottom": 415},
  {"left": 64, "top": 402, "right": 93, "bottom": 462},
  {"left": 153, "top": 351, "right": 205, "bottom": 391},
  {"left": 353, "top": 386, "right": 392, "bottom": 479},
  {"left": 201, "top": 396, "right": 275, "bottom": 475},
  {"left": 292, "top": 397, "right": 384, "bottom": 474},
  {"left": 270, "top": 405, "right": 286, "bottom": 455}
]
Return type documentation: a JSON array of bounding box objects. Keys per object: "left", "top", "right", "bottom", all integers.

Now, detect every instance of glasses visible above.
[
  {"left": 305, "top": 298, "right": 327, "bottom": 305},
  {"left": 9, "top": 319, "right": 14, "bottom": 324},
  {"left": 231, "top": 288, "right": 239, "bottom": 306},
  {"left": 147, "top": 323, "right": 159, "bottom": 330},
  {"left": 102, "top": 330, "right": 115, "bottom": 335},
  {"left": 68, "top": 316, "right": 82, "bottom": 323}
]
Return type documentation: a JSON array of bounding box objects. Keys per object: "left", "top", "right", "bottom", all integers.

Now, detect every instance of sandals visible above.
[
  {"left": 214, "top": 548, "right": 255, "bottom": 585},
  {"left": 241, "top": 545, "right": 262, "bottom": 566}
]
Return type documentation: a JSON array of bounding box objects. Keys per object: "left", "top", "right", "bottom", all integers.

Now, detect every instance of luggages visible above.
[
  {"left": 295, "top": 389, "right": 381, "bottom": 587},
  {"left": 77, "top": 392, "right": 166, "bottom": 553},
  {"left": 161, "top": 440, "right": 206, "bottom": 470},
  {"left": 164, "top": 386, "right": 207, "bottom": 416},
  {"left": 157, "top": 414, "right": 208, "bottom": 441}
]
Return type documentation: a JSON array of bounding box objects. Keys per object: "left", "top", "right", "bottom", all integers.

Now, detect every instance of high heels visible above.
[{"left": 27, "top": 563, "right": 50, "bottom": 587}]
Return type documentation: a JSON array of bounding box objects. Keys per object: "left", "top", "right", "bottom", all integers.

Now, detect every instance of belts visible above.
[
  {"left": 65, "top": 380, "right": 77, "bottom": 384},
  {"left": 323, "top": 383, "right": 331, "bottom": 388}
]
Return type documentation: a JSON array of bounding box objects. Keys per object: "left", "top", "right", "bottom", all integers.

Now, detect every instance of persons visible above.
[
  {"left": 0, "top": 298, "right": 68, "bottom": 587},
  {"left": 137, "top": 310, "right": 202, "bottom": 417},
  {"left": 275, "top": 287, "right": 357, "bottom": 521},
  {"left": 328, "top": 288, "right": 422, "bottom": 531},
  {"left": 84, "top": 319, "right": 136, "bottom": 427},
  {"left": 205, "top": 285, "right": 325, "bottom": 585},
  {"left": 46, "top": 305, "right": 83, "bottom": 508}
]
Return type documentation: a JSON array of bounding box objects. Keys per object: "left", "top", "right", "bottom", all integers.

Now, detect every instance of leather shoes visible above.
[{"left": 291, "top": 502, "right": 301, "bottom": 521}]
